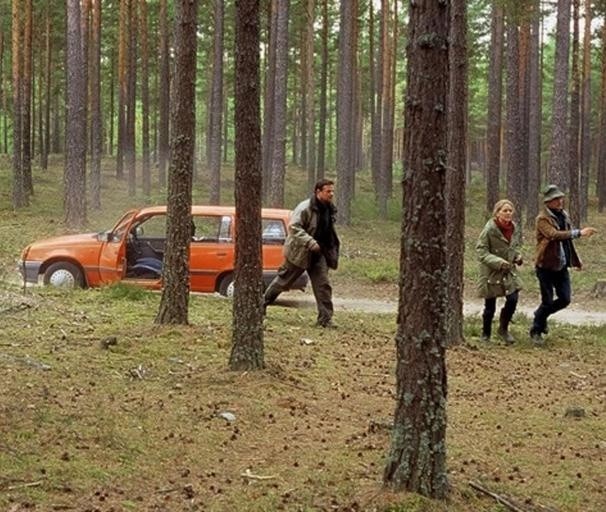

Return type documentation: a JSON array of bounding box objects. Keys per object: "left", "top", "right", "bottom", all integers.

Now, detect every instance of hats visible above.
[{"left": 543, "top": 185, "right": 565, "bottom": 202}]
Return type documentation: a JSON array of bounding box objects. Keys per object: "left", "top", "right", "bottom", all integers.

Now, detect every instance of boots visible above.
[
  {"left": 481, "top": 315, "right": 491, "bottom": 340},
  {"left": 498, "top": 309, "right": 513, "bottom": 342}
]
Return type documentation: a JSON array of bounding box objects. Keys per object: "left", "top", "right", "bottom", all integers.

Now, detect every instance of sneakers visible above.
[
  {"left": 319, "top": 320, "right": 338, "bottom": 328},
  {"left": 530, "top": 312, "right": 548, "bottom": 338}
]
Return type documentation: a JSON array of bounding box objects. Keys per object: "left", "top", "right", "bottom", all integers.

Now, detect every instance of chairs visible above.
[{"left": 133, "top": 258, "right": 161, "bottom": 278}]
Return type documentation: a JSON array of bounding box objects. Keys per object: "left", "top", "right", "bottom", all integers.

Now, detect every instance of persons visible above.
[
  {"left": 259, "top": 179, "right": 342, "bottom": 330},
  {"left": 475, "top": 199, "right": 524, "bottom": 345},
  {"left": 529, "top": 183, "right": 600, "bottom": 340}
]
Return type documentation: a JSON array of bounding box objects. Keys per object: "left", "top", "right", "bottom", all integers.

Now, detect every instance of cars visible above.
[{"left": 18, "top": 205, "right": 310, "bottom": 292}]
